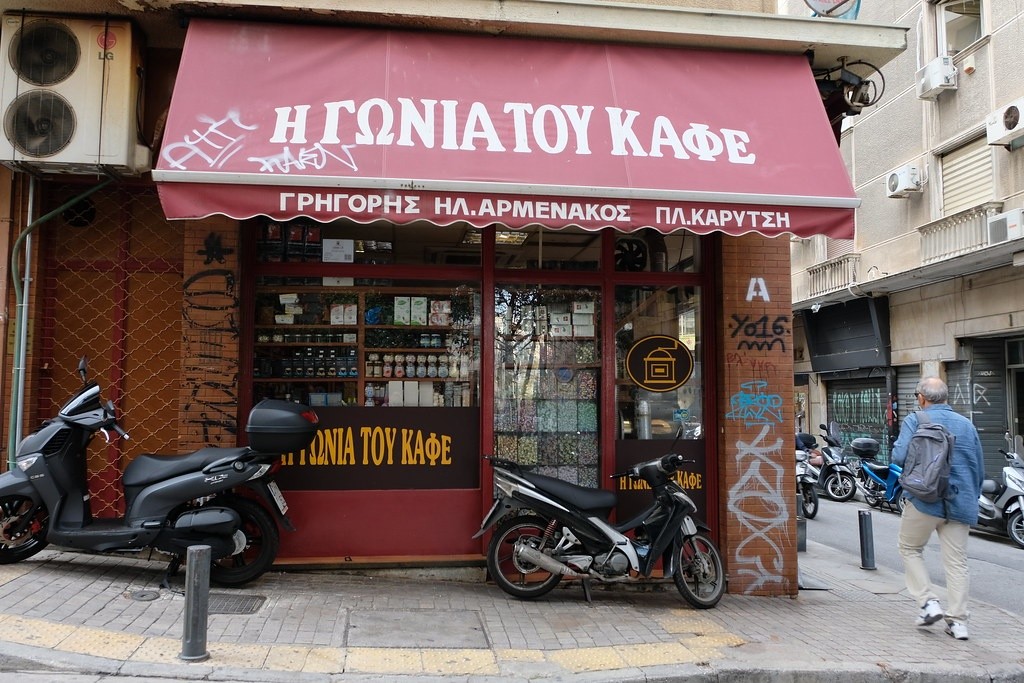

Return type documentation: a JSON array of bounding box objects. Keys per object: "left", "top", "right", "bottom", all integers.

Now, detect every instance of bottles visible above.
[
  {"left": 284, "top": 333, "right": 343, "bottom": 343},
  {"left": 266, "top": 363, "right": 272, "bottom": 376},
  {"left": 254, "top": 368, "right": 259, "bottom": 378},
  {"left": 284, "top": 394, "right": 291, "bottom": 401},
  {"left": 260, "top": 358, "right": 265, "bottom": 376},
  {"left": 282, "top": 348, "right": 358, "bottom": 378}
]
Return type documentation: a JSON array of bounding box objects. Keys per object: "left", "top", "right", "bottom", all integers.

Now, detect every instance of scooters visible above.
[
  {"left": 969, "top": 446, "right": 1024, "bottom": 550},
  {"left": 0, "top": 356, "right": 321, "bottom": 588},
  {"left": 797, "top": 421, "right": 856, "bottom": 501},
  {"left": 851, "top": 438, "right": 908, "bottom": 515},
  {"left": 796, "top": 450, "right": 820, "bottom": 519}
]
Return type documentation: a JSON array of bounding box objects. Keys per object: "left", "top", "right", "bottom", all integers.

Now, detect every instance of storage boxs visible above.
[
  {"left": 326, "top": 392, "right": 343, "bottom": 407},
  {"left": 431, "top": 300, "right": 451, "bottom": 314},
  {"left": 428, "top": 313, "right": 450, "bottom": 325},
  {"left": 285, "top": 304, "right": 304, "bottom": 315},
  {"left": 411, "top": 296, "right": 428, "bottom": 325},
  {"left": 343, "top": 333, "right": 356, "bottom": 343},
  {"left": 330, "top": 303, "right": 344, "bottom": 325},
  {"left": 275, "top": 314, "right": 295, "bottom": 324},
  {"left": 404, "top": 380, "right": 419, "bottom": 406},
  {"left": 420, "top": 382, "right": 434, "bottom": 406},
  {"left": 389, "top": 381, "right": 403, "bottom": 406},
  {"left": 278, "top": 293, "right": 299, "bottom": 305},
  {"left": 344, "top": 303, "right": 357, "bottom": 326},
  {"left": 257, "top": 306, "right": 274, "bottom": 325},
  {"left": 308, "top": 393, "right": 326, "bottom": 406},
  {"left": 628, "top": 302, "right": 679, "bottom": 341},
  {"left": 393, "top": 296, "right": 410, "bottom": 325}
]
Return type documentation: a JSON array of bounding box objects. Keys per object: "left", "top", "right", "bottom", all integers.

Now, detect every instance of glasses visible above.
[{"left": 914, "top": 392, "right": 924, "bottom": 397}]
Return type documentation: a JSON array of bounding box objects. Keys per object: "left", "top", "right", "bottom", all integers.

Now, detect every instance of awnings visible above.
[{"left": 151, "top": 19, "right": 862, "bottom": 239}]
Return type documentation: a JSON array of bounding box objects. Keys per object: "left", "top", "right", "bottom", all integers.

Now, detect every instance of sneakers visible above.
[
  {"left": 945, "top": 621, "right": 968, "bottom": 639},
  {"left": 916, "top": 601, "right": 944, "bottom": 626}
]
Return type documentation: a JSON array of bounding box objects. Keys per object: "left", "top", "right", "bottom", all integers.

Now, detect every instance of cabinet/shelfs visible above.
[{"left": 254, "top": 286, "right": 602, "bottom": 407}]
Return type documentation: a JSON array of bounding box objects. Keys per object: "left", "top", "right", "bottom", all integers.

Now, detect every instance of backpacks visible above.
[{"left": 899, "top": 412, "right": 956, "bottom": 503}]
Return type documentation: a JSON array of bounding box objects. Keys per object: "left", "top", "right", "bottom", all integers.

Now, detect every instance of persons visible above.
[
  {"left": 795, "top": 433, "right": 809, "bottom": 453},
  {"left": 891, "top": 377, "right": 984, "bottom": 639}
]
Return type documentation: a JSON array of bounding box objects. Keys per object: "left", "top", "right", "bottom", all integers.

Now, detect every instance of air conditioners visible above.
[
  {"left": 986, "top": 207, "right": 1024, "bottom": 246},
  {"left": 886, "top": 164, "right": 920, "bottom": 195},
  {"left": 985, "top": 96, "right": 1024, "bottom": 144},
  {"left": 914, "top": 55, "right": 955, "bottom": 98},
  {"left": 0, "top": 15, "right": 146, "bottom": 179}
]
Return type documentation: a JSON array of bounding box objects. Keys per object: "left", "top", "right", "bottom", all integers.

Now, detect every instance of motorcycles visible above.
[{"left": 472, "top": 426, "right": 728, "bottom": 610}]
